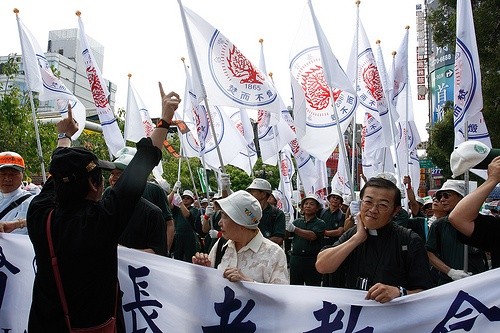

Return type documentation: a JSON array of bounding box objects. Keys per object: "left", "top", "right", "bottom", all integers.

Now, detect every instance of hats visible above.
[
  {"left": 407, "top": 198, "right": 424, "bottom": 209},
  {"left": 0, "top": 152, "right": 26, "bottom": 172},
  {"left": 436, "top": 180, "right": 464, "bottom": 198},
  {"left": 271, "top": 189, "right": 282, "bottom": 202},
  {"left": 372, "top": 173, "right": 397, "bottom": 184},
  {"left": 326, "top": 190, "right": 343, "bottom": 203},
  {"left": 421, "top": 196, "right": 433, "bottom": 211},
  {"left": 201, "top": 198, "right": 208, "bottom": 205},
  {"left": 214, "top": 189, "right": 262, "bottom": 229},
  {"left": 450, "top": 141, "right": 500, "bottom": 179},
  {"left": 111, "top": 147, "right": 137, "bottom": 168},
  {"left": 182, "top": 190, "right": 195, "bottom": 203},
  {"left": 300, "top": 194, "right": 323, "bottom": 211},
  {"left": 247, "top": 178, "right": 272, "bottom": 195},
  {"left": 50, "top": 146, "right": 116, "bottom": 184}
]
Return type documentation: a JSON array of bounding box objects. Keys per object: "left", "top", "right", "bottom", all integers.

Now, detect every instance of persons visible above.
[{"left": 0, "top": 81, "right": 500, "bottom": 333}]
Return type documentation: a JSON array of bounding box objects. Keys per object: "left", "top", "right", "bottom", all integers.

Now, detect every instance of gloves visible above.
[
  {"left": 284, "top": 212, "right": 295, "bottom": 233},
  {"left": 172, "top": 193, "right": 183, "bottom": 208},
  {"left": 203, "top": 207, "right": 214, "bottom": 220},
  {"left": 173, "top": 181, "right": 182, "bottom": 192},
  {"left": 208, "top": 230, "right": 222, "bottom": 238},
  {"left": 447, "top": 268, "right": 472, "bottom": 280}
]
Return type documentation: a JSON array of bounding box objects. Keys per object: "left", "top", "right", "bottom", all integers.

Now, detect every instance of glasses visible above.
[
  {"left": 436, "top": 191, "right": 456, "bottom": 200},
  {"left": 359, "top": 198, "right": 393, "bottom": 212},
  {"left": 424, "top": 205, "right": 432, "bottom": 209},
  {"left": 181, "top": 196, "right": 190, "bottom": 200}
]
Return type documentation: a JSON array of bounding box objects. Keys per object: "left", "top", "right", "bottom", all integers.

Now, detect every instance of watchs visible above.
[
  {"left": 397, "top": 286, "right": 407, "bottom": 297},
  {"left": 156, "top": 119, "right": 169, "bottom": 129},
  {"left": 57, "top": 133, "right": 71, "bottom": 140},
  {"left": 16, "top": 217, "right": 23, "bottom": 229}
]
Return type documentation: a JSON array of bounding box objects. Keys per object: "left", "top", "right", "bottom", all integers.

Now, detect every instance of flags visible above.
[
  {"left": 454, "top": 0, "right": 492, "bottom": 149},
  {"left": 126, "top": 79, "right": 164, "bottom": 176},
  {"left": 18, "top": 18, "right": 86, "bottom": 141},
  {"left": 78, "top": 19, "right": 125, "bottom": 158},
  {"left": 181, "top": 3, "right": 421, "bottom": 222}
]
